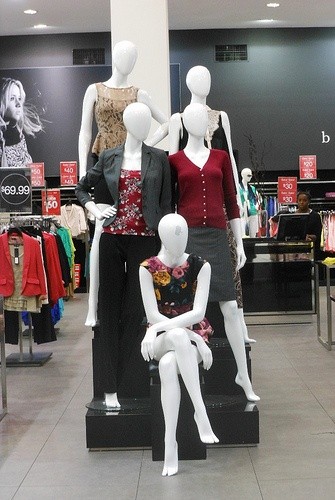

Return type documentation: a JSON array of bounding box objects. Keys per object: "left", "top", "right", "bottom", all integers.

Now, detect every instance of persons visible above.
[
  {"left": 293, "top": 192, "right": 322, "bottom": 259},
  {"left": 138, "top": 214, "right": 220, "bottom": 476},
  {"left": 237, "top": 167, "right": 262, "bottom": 238},
  {"left": 170, "top": 65, "right": 256, "bottom": 343},
  {"left": 0, "top": 77, "right": 45, "bottom": 167},
  {"left": 168, "top": 103, "right": 260, "bottom": 401},
  {"left": 75, "top": 103, "right": 173, "bottom": 408},
  {"left": 78, "top": 41, "right": 170, "bottom": 326}
]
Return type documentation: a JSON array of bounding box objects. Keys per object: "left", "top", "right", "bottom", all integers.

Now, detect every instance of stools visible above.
[{"left": 150, "top": 360, "right": 207, "bottom": 461}]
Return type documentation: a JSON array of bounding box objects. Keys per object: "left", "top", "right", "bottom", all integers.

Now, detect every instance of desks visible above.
[{"left": 314, "top": 260, "right": 335, "bottom": 351}]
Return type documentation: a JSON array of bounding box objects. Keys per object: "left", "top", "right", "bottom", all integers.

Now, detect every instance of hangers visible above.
[
  {"left": 65, "top": 198, "right": 73, "bottom": 206},
  {"left": 318, "top": 209, "right": 335, "bottom": 218},
  {"left": 1, "top": 218, "right": 62, "bottom": 239}
]
